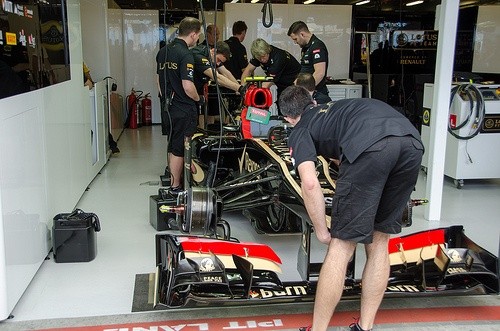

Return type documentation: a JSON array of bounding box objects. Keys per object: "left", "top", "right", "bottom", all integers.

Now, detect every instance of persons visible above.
[
  {"left": 156, "top": 17, "right": 204, "bottom": 198},
  {"left": 241, "top": 38, "right": 300, "bottom": 117},
  {"left": 199, "top": 25, "right": 223, "bottom": 132},
  {"left": 190, "top": 41, "right": 244, "bottom": 105},
  {"left": 296, "top": 74, "right": 332, "bottom": 103},
  {"left": 223, "top": 20, "right": 249, "bottom": 111},
  {"left": 287, "top": 20, "right": 332, "bottom": 94},
  {"left": 279, "top": 86, "right": 426, "bottom": 330},
  {"left": 83, "top": 59, "right": 121, "bottom": 155}
]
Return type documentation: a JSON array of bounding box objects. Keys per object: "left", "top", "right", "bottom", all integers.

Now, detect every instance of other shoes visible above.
[{"left": 169, "top": 185, "right": 184, "bottom": 194}]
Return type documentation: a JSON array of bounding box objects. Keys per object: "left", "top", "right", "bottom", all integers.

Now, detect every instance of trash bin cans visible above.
[{"left": 52, "top": 212, "right": 97, "bottom": 262}]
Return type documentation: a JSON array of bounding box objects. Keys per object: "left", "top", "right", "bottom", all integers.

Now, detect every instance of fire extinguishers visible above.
[
  {"left": 138, "top": 92, "right": 152, "bottom": 125},
  {"left": 125, "top": 88, "right": 144, "bottom": 129},
  {"left": 110, "top": 90, "right": 123, "bottom": 129}
]
